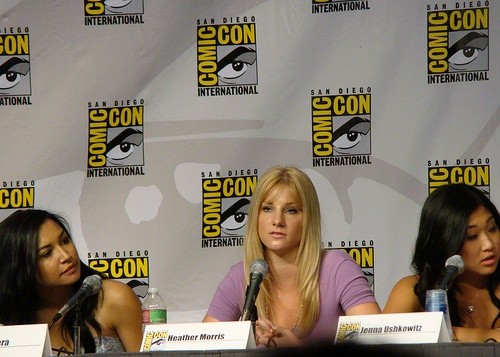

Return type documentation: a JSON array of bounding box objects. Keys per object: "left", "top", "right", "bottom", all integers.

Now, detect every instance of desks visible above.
[{"left": 87, "top": 342, "right": 500, "bottom": 357}]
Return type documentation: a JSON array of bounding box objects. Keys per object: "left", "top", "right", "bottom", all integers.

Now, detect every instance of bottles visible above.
[{"left": 141, "top": 288, "right": 167, "bottom": 337}]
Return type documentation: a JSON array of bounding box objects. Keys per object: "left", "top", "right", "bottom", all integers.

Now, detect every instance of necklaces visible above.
[{"left": 457, "top": 288, "right": 487, "bottom": 315}]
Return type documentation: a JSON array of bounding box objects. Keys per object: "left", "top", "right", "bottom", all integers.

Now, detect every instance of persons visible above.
[
  {"left": 380, "top": 182, "right": 499, "bottom": 344},
  {"left": 0, "top": 210, "right": 143, "bottom": 357},
  {"left": 200, "top": 164, "right": 382, "bottom": 351}
]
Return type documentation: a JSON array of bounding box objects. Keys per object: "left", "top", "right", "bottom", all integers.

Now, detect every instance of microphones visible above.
[
  {"left": 52, "top": 274, "right": 102, "bottom": 323},
  {"left": 242, "top": 259, "right": 269, "bottom": 321},
  {"left": 440, "top": 255, "right": 465, "bottom": 292}
]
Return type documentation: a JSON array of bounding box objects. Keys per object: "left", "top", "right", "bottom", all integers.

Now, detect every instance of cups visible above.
[{"left": 425, "top": 290, "right": 453, "bottom": 343}]
